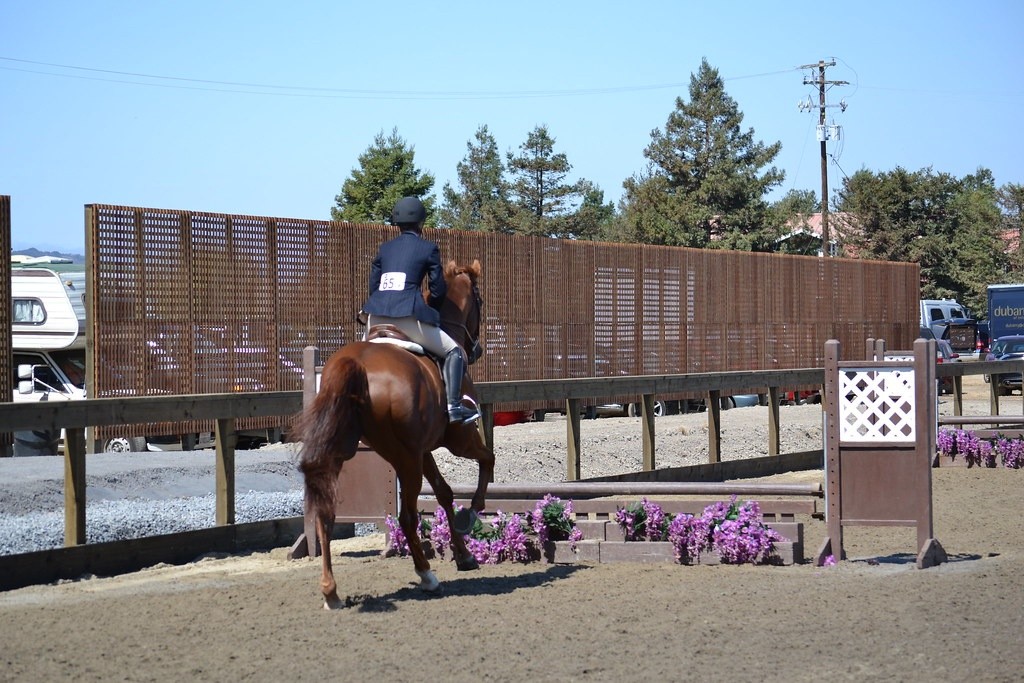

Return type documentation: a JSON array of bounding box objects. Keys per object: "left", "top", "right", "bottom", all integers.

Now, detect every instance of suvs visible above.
[{"left": 983, "top": 333, "right": 1024, "bottom": 383}]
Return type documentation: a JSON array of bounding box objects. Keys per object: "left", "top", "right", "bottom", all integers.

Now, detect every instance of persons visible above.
[{"left": 355, "top": 198, "right": 478, "bottom": 423}]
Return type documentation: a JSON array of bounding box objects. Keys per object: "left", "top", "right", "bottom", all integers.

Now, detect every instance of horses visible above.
[{"left": 298, "top": 258, "right": 497, "bottom": 612}]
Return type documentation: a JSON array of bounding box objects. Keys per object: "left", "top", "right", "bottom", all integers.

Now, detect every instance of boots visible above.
[{"left": 443, "top": 346, "right": 478, "bottom": 423}]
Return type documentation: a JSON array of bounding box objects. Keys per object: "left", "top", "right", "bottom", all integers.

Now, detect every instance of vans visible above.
[{"left": 919, "top": 298, "right": 972, "bottom": 329}]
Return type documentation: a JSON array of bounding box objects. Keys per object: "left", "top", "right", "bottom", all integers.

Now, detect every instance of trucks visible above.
[
  {"left": 9, "top": 253, "right": 364, "bottom": 456},
  {"left": 986, "top": 284, "right": 1024, "bottom": 351}
]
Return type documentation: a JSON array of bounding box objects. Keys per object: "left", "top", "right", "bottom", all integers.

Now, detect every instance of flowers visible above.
[
  {"left": 937, "top": 428, "right": 1024, "bottom": 469},
  {"left": 615, "top": 494, "right": 787, "bottom": 565},
  {"left": 383, "top": 493, "right": 582, "bottom": 565}
]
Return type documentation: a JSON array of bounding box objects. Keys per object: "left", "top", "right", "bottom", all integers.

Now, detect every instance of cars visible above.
[
  {"left": 919, "top": 323, "right": 959, "bottom": 397},
  {"left": 475, "top": 315, "right": 825, "bottom": 430},
  {"left": 995, "top": 352, "right": 1024, "bottom": 397}
]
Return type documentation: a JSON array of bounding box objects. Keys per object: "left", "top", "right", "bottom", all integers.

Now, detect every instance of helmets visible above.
[{"left": 392, "top": 197, "right": 427, "bottom": 223}]
